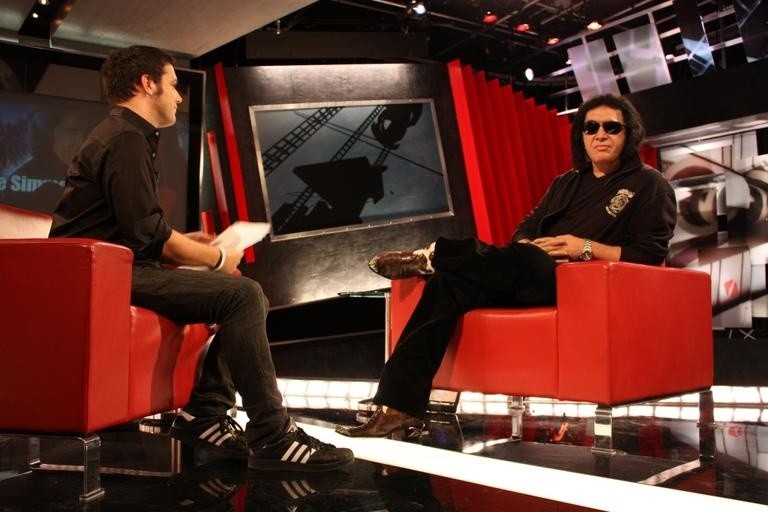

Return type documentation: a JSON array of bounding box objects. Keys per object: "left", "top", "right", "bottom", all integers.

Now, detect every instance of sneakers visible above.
[
  {"left": 246, "top": 415, "right": 354, "bottom": 473},
  {"left": 169, "top": 403, "right": 249, "bottom": 456}
]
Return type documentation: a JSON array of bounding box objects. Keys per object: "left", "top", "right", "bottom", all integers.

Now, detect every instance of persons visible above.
[
  {"left": 335, "top": 92, "right": 678, "bottom": 438},
  {"left": 47, "top": 44, "right": 357, "bottom": 472}
]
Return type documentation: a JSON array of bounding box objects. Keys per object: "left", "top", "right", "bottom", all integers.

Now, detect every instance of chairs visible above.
[
  {"left": 0, "top": 237, "right": 221, "bottom": 504},
  {"left": 388, "top": 259, "right": 714, "bottom": 457}
]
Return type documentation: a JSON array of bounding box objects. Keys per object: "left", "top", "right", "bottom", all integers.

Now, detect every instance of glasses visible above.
[{"left": 577, "top": 120, "right": 626, "bottom": 135}]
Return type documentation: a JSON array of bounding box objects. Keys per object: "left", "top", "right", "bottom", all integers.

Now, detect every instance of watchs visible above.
[{"left": 581, "top": 238, "right": 593, "bottom": 261}]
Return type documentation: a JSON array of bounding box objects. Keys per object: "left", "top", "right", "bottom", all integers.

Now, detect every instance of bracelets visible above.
[{"left": 208, "top": 247, "right": 226, "bottom": 272}]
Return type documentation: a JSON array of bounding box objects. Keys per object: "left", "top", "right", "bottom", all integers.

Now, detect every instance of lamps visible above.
[
  {"left": 480, "top": 0, "right": 499, "bottom": 25},
  {"left": 540, "top": 25, "right": 561, "bottom": 46},
  {"left": 412, "top": 4, "right": 428, "bottom": 18},
  {"left": 585, "top": 11, "right": 604, "bottom": 31},
  {"left": 509, "top": 14, "right": 530, "bottom": 33},
  {"left": 515, "top": 66, "right": 535, "bottom": 84},
  {"left": 665, "top": 45, "right": 676, "bottom": 60}
]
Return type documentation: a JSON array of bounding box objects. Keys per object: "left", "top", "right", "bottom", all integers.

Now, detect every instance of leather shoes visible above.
[
  {"left": 368, "top": 248, "right": 433, "bottom": 280},
  {"left": 334, "top": 404, "right": 423, "bottom": 437}
]
